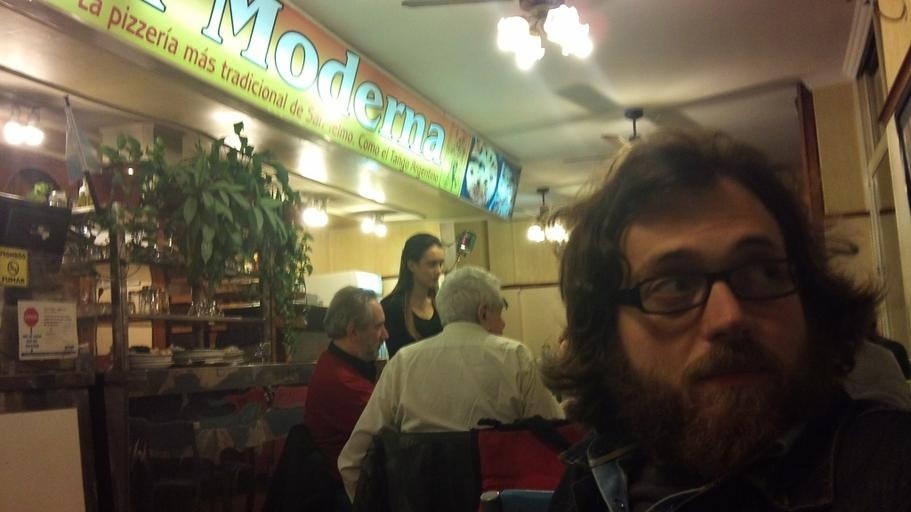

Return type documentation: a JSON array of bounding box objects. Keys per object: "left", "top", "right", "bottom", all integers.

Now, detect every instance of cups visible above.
[
  {"left": 189, "top": 298, "right": 225, "bottom": 316},
  {"left": 126, "top": 285, "right": 171, "bottom": 315}
]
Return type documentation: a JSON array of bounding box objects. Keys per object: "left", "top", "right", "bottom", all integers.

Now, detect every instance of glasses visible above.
[{"left": 612, "top": 254, "right": 807, "bottom": 316}]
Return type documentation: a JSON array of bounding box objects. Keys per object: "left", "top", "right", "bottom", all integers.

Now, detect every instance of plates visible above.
[
  {"left": 466, "top": 141, "right": 499, "bottom": 205},
  {"left": 131, "top": 348, "right": 253, "bottom": 369}
]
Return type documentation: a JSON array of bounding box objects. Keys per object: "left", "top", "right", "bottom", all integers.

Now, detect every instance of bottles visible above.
[{"left": 45, "top": 188, "right": 68, "bottom": 210}]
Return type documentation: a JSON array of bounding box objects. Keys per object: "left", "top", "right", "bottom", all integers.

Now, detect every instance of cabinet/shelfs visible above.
[{"left": 65, "top": 256, "right": 244, "bottom": 362}]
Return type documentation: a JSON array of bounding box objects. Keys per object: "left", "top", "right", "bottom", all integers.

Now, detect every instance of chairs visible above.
[{"left": 281, "top": 421, "right": 481, "bottom": 512}]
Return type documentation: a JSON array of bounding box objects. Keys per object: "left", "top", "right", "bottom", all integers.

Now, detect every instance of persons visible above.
[
  {"left": 335, "top": 265, "right": 567, "bottom": 502},
  {"left": 533, "top": 122, "right": 911, "bottom": 511},
  {"left": 304, "top": 284, "right": 391, "bottom": 465},
  {"left": 381, "top": 232, "right": 445, "bottom": 360}
]
